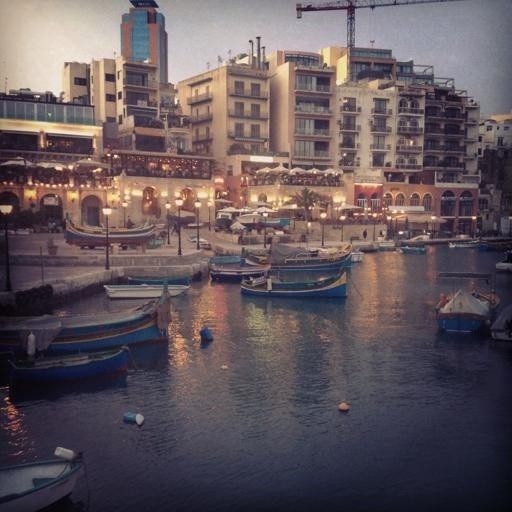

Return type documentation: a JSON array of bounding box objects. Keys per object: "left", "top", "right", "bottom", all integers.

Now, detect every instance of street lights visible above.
[
  {"left": 207, "top": 201, "right": 213, "bottom": 231},
  {"left": 107, "top": 152, "right": 118, "bottom": 176},
  {"left": 332, "top": 190, "right": 341, "bottom": 228},
  {"left": 165, "top": 200, "right": 171, "bottom": 244},
  {"left": 263, "top": 211, "right": 269, "bottom": 250},
  {"left": 176, "top": 197, "right": 184, "bottom": 255},
  {"left": 103, "top": 203, "right": 113, "bottom": 269},
  {"left": 320, "top": 212, "right": 327, "bottom": 247},
  {"left": 0, "top": 198, "right": 16, "bottom": 291},
  {"left": 122, "top": 200, "right": 129, "bottom": 227},
  {"left": 352, "top": 204, "right": 478, "bottom": 241},
  {"left": 339, "top": 215, "right": 346, "bottom": 243},
  {"left": 195, "top": 200, "right": 202, "bottom": 249}
]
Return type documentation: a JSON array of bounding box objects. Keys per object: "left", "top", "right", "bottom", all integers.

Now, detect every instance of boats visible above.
[
  {"left": 237, "top": 213, "right": 261, "bottom": 228},
  {"left": 434, "top": 272, "right": 502, "bottom": 339},
  {"left": 362, "top": 237, "right": 512, "bottom": 270},
  {"left": 259, "top": 217, "right": 289, "bottom": 229},
  {"left": 0, "top": 437, "right": 85, "bottom": 512},
  {"left": 215, "top": 209, "right": 234, "bottom": 229},
  {"left": 0, "top": 277, "right": 171, "bottom": 356},
  {"left": 64, "top": 214, "right": 157, "bottom": 249},
  {"left": 491, "top": 303, "right": 511, "bottom": 341},
  {"left": 103, "top": 282, "right": 190, "bottom": 300},
  {"left": 206, "top": 241, "right": 363, "bottom": 301},
  {"left": 5, "top": 344, "right": 131, "bottom": 395}
]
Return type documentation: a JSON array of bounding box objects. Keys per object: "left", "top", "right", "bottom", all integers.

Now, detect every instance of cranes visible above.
[{"left": 297, "top": 1, "right": 457, "bottom": 51}]
